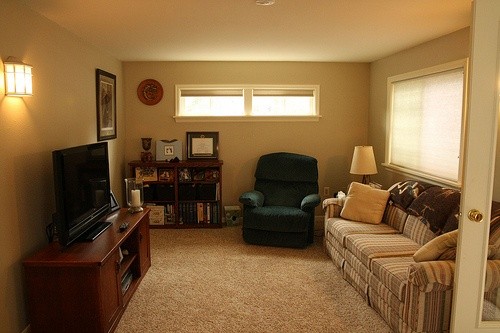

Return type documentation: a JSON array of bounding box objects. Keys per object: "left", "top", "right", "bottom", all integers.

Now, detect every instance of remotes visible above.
[{"left": 120, "top": 222, "right": 129, "bottom": 230}]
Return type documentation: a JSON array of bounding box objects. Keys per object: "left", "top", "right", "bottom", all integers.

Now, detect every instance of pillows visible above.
[
  {"left": 339, "top": 182, "right": 390, "bottom": 225},
  {"left": 412, "top": 228, "right": 458, "bottom": 263}
]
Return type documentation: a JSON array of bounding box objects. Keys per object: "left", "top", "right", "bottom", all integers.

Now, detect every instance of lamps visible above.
[
  {"left": 3, "top": 56, "right": 34, "bottom": 98},
  {"left": 349, "top": 145, "right": 378, "bottom": 184}
]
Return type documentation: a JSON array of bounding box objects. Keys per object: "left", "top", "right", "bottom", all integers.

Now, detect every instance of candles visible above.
[{"left": 131, "top": 189, "right": 140, "bottom": 207}]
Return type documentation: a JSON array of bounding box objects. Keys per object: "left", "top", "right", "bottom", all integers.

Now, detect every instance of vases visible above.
[{"left": 139, "top": 137, "right": 156, "bottom": 162}]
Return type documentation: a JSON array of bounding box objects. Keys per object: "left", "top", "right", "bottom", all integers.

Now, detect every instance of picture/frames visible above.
[
  {"left": 155, "top": 139, "right": 183, "bottom": 161},
  {"left": 96, "top": 68, "right": 117, "bottom": 142}
]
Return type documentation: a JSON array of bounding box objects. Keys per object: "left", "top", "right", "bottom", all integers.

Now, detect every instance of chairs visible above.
[{"left": 238, "top": 152, "right": 322, "bottom": 250}]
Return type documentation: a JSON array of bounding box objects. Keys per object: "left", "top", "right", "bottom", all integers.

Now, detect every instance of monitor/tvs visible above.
[{"left": 52, "top": 142, "right": 113, "bottom": 247}]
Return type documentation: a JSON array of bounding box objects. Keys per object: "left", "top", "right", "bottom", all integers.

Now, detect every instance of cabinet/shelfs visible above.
[
  {"left": 127, "top": 160, "right": 224, "bottom": 229},
  {"left": 20, "top": 207, "right": 152, "bottom": 333}
]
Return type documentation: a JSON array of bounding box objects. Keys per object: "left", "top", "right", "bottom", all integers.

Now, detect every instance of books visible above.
[{"left": 143, "top": 183, "right": 221, "bottom": 225}]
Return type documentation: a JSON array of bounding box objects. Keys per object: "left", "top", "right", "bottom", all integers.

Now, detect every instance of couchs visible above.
[{"left": 321, "top": 179, "right": 500, "bottom": 333}]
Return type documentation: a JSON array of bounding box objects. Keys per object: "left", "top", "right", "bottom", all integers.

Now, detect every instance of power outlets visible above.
[{"left": 323, "top": 187, "right": 329, "bottom": 196}]
